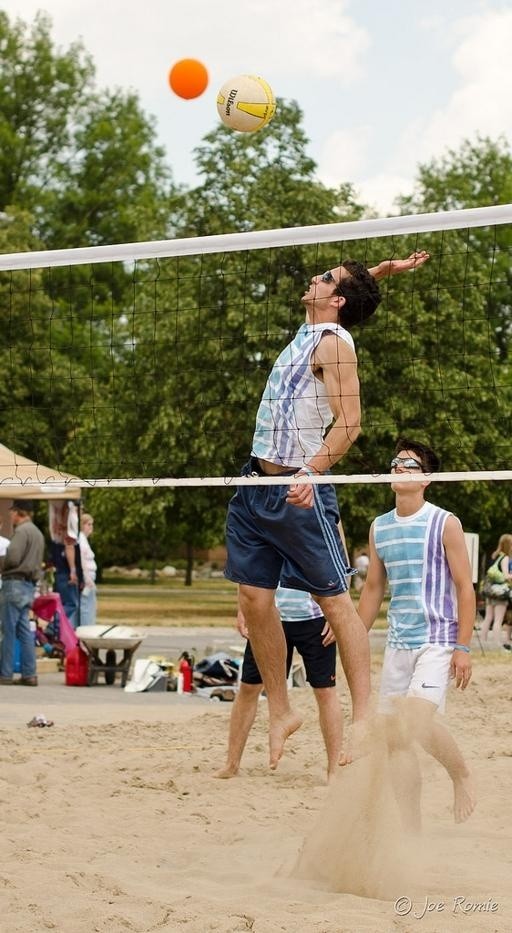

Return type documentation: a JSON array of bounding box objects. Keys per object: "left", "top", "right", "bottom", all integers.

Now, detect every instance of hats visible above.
[{"left": 9, "top": 496, "right": 32, "bottom": 512}]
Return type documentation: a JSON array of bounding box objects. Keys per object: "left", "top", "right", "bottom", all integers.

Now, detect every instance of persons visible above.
[
  {"left": 356, "top": 436, "right": 480, "bottom": 837},
  {"left": 0, "top": 498, "right": 46, "bottom": 686},
  {"left": 477, "top": 532, "right": 511, "bottom": 647},
  {"left": 48, "top": 517, "right": 79, "bottom": 628},
  {"left": 79, "top": 513, "right": 98, "bottom": 627},
  {"left": 354, "top": 550, "right": 370, "bottom": 580},
  {"left": 210, "top": 505, "right": 353, "bottom": 784},
  {"left": 224, "top": 247, "right": 430, "bottom": 772}
]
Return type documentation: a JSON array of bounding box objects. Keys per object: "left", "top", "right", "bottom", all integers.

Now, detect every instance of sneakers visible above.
[{"left": 0, "top": 675, "right": 38, "bottom": 687}]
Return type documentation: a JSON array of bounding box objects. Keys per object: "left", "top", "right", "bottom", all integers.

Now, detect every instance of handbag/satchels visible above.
[{"left": 202, "top": 659, "right": 239, "bottom": 682}]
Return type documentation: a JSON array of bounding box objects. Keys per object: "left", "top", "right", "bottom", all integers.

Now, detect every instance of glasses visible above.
[
  {"left": 390, "top": 456, "right": 422, "bottom": 470},
  {"left": 322, "top": 270, "right": 347, "bottom": 296}
]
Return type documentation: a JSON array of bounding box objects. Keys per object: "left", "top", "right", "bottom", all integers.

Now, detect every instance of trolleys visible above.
[{"left": 74, "top": 624, "right": 147, "bottom": 687}]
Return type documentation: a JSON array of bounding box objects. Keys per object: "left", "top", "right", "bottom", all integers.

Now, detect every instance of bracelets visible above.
[
  {"left": 300, "top": 466, "right": 313, "bottom": 476},
  {"left": 454, "top": 642, "right": 472, "bottom": 654}
]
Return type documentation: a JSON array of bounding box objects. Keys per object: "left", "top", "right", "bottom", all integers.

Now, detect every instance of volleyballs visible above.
[
  {"left": 169, "top": 58, "right": 207, "bottom": 99},
  {"left": 216, "top": 74, "right": 276, "bottom": 132}
]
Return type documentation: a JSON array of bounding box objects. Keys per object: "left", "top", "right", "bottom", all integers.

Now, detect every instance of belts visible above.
[{"left": 1, "top": 573, "right": 37, "bottom": 581}]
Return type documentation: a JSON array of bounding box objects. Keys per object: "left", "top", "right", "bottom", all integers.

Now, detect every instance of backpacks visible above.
[{"left": 486, "top": 553, "right": 507, "bottom": 585}]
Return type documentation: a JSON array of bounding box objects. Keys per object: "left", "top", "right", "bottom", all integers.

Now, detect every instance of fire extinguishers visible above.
[{"left": 178, "top": 651, "right": 193, "bottom": 692}]
